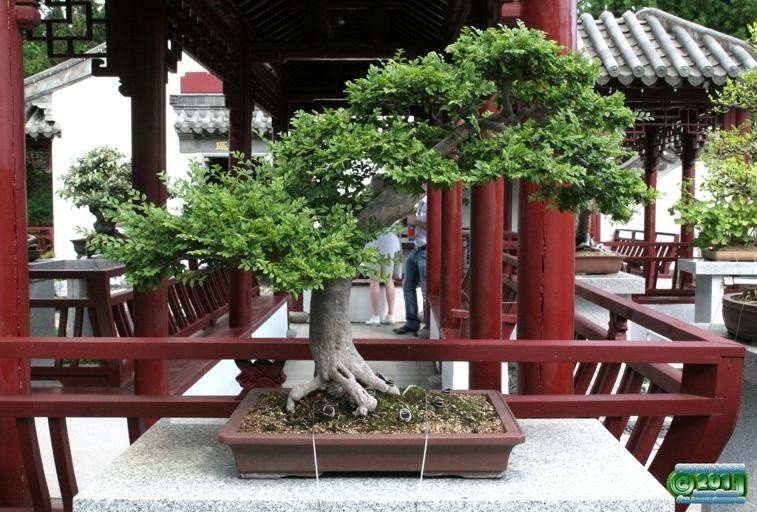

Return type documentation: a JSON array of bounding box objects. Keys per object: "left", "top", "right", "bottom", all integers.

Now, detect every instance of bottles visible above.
[{"left": 408, "top": 223, "right": 415, "bottom": 242}]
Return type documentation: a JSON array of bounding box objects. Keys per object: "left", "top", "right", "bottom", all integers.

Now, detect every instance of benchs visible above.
[{"left": 622, "top": 261, "right": 663, "bottom": 277}]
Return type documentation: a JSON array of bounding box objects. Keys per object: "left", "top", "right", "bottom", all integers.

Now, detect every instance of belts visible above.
[{"left": 413, "top": 244, "right": 427, "bottom": 253}]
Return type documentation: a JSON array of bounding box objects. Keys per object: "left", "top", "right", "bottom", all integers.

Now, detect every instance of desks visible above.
[{"left": 69, "top": 416, "right": 676, "bottom": 512}]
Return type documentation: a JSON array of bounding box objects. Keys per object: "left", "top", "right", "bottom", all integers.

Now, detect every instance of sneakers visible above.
[
  {"left": 381, "top": 314, "right": 395, "bottom": 326},
  {"left": 365, "top": 315, "right": 380, "bottom": 327},
  {"left": 392, "top": 325, "right": 419, "bottom": 334}
]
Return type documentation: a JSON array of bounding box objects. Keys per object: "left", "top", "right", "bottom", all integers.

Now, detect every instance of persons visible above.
[
  {"left": 392, "top": 197, "right": 428, "bottom": 336},
  {"left": 363, "top": 175, "right": 401, "bottom": 326}
]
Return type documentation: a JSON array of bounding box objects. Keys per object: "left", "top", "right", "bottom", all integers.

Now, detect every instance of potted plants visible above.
[
  {"left": 84, "top": 16, "right": 661, "bottom": 481},
  {"left": 55, "top": 148, "right": 176, "bottom": 260},
  {"left": 667, "top": 20, "right": 757, "bottom": 260}
]
